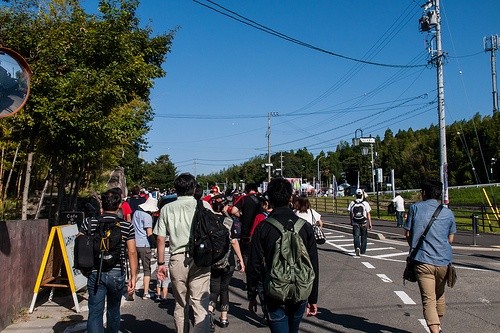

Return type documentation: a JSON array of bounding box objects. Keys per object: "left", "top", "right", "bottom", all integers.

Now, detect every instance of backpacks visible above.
[
  {"left": 266, "top": 218, "right": 316, "bottom": 301},
  {"left": 117, "top": 202, "right": 124, "bottom": 219},
  {"left": 94, "top": 218, "right": 125, "bottom": 272},
  {"left": 187, "top": 200, "right": 230, "bottom": 266},
  {"left": 212, "top": 215, "right": 227, "bottom": 266},
  {"left": 350, "top": 200, "right": 367, "bottom": 224}
]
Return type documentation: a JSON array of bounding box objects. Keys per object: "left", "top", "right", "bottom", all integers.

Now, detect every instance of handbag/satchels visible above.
[
  {"left": 403, "top": 257, "right": 418, "bottom": 282},
  {"left": 230, "top": 217, "right": 241, "bottom": 239},
  {"left": 447, "top": 263, "right": 457, "bottom": 287},
  {"left": 310, "top": 209, "right": 326, "bottom": 245},
  {"left": 388, "top": 204, "right": 396, "bottom": 214},
  {"left": 74, "top": 218, "right": 94, "bottom": 269}
]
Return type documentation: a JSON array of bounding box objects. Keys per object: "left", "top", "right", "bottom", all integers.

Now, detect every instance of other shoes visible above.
[
  {"left": 355, "top": 247, "right": 360, "bottom": 256},
  {"left": 155, "top": 295, "right": 161, "bottom": 300},
  {"left": 128, "top": 287, "right": 134, "bottom": 301},
  {"left": 142, "top": 294, "right": 151, "bottom": 299},
  {"left": 208, "top": 311, "right": 216, "bottom": 333},
  {"left": 219, "top": 317, "right": 229, "bottom": 327}
]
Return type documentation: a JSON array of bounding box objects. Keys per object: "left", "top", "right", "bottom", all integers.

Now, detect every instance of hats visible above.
[
  {"left": 356, "top": 188, "right": 363, "bottom": 195},
  {"left": 139, "top": 197, "right": 159, "bottom": 212},
  {"left": 211, "top": 186, "right": 222, "bottom": 194}
]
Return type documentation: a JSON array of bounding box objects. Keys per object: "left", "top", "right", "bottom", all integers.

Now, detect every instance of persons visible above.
[
  {"left": 204, "top": 184, "right": 272, "bottom": 327},
  {"left": 101, "top": 187, "right": 131, "bottom": 222},
  {"left": 247, "top": 177, "right": 319, "bottom": 333},
  {"left": 403, "top": 178, "right": 456, "bottom": 333},
  {"left": 77, "top": 189, "right": 137, "bottom": 333},
  {"left": 390, "top": 192, "right": 405, "bottom": 228},
  {"left": 348, "top": 188, "right": 372, "bottom": 256},
  {"left": 153, "top": 173, "right": 215, "bottom": 333},
  {"left": 295, "top": 194, "right": 322, "bottom": 229},
  {"left": 126, "top": 186, "right": 172, "bottom": 302}
]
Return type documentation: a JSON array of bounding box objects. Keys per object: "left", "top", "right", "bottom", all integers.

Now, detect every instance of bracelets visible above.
[{"left": 158, "top": 262, "right": 165, "bottom": 265}]
